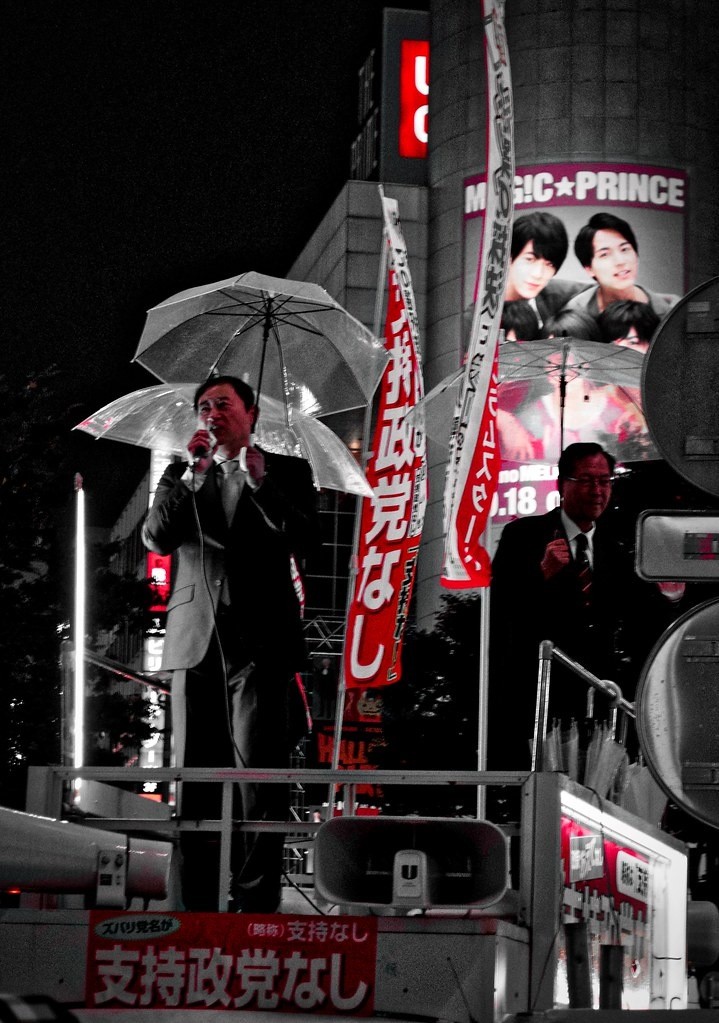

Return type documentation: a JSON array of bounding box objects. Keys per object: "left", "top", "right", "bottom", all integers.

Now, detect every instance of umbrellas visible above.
[
  {"left": 408, "top": 332, "right": 668, "bottom": 465},
  {"left": 527, "top": 677, "right": 668, "bottom": 825},
  {"left": 132, "top": 269, "right": 394, "bottom": 418},
  {"left": 71, "top": 382, "right": 377, "bottom": 504}
]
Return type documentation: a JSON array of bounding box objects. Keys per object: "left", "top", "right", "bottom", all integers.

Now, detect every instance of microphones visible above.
[{"left": 188, "top": 427, "right": 218, "bottom": 471}]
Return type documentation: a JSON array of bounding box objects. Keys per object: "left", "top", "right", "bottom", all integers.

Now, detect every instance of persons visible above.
[
  {"left": 492, "top": 442, "right": 617, "bottom": 785},
  {"left": 140, "top": 374, "right": 319, "bottom": 913},
  {"left": 469, "top": 212, "right": 682, "bottom": 357}
]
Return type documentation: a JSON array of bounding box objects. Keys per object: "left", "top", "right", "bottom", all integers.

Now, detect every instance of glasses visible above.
[{"left": 561, "top": 474, "right": 615, "bottom": 490}]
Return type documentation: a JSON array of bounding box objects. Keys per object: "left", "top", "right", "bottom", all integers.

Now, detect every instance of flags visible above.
[{"left": 337, "top": 193, "right": 424, "bottom": 689}]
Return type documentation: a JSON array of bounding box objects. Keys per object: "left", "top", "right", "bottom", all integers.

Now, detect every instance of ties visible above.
[
  {"left": 573, "top": 533, "right": 595, "bottom": 621},
  {"left": 217, "top": 461, "right": 240, "bottom": 530}
]
